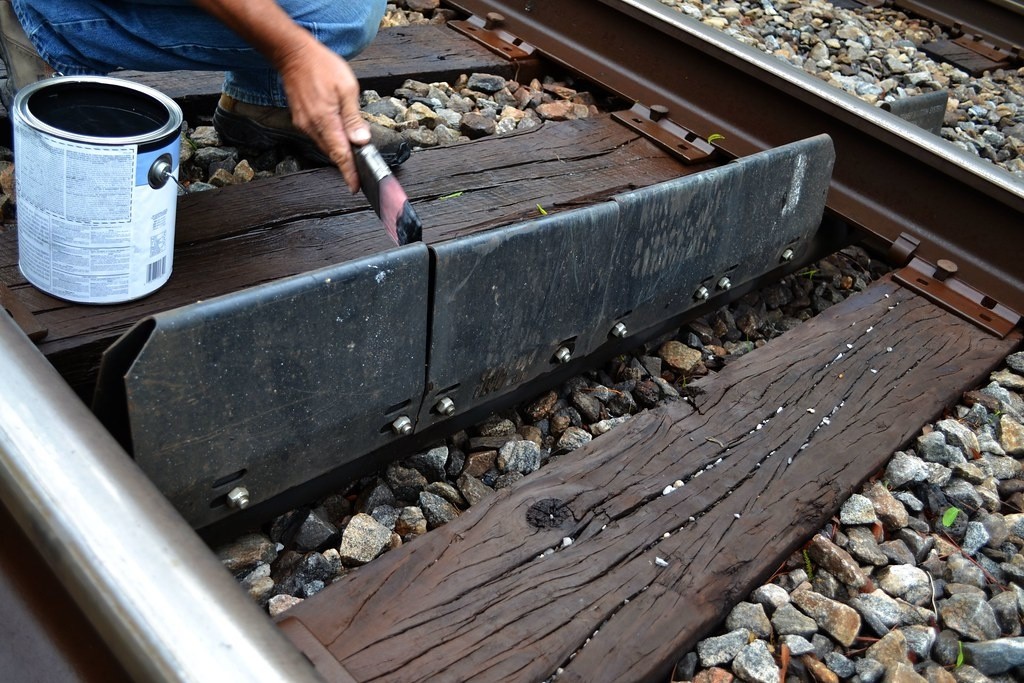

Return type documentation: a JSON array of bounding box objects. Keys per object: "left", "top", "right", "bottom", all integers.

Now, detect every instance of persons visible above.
[{"left": 0, "top": 0, "right": 411, "bottom": 195}]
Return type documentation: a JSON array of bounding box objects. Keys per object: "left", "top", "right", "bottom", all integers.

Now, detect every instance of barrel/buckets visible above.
[{"left": 12, "top": 76, "right": 185, "bottom": 302}]
